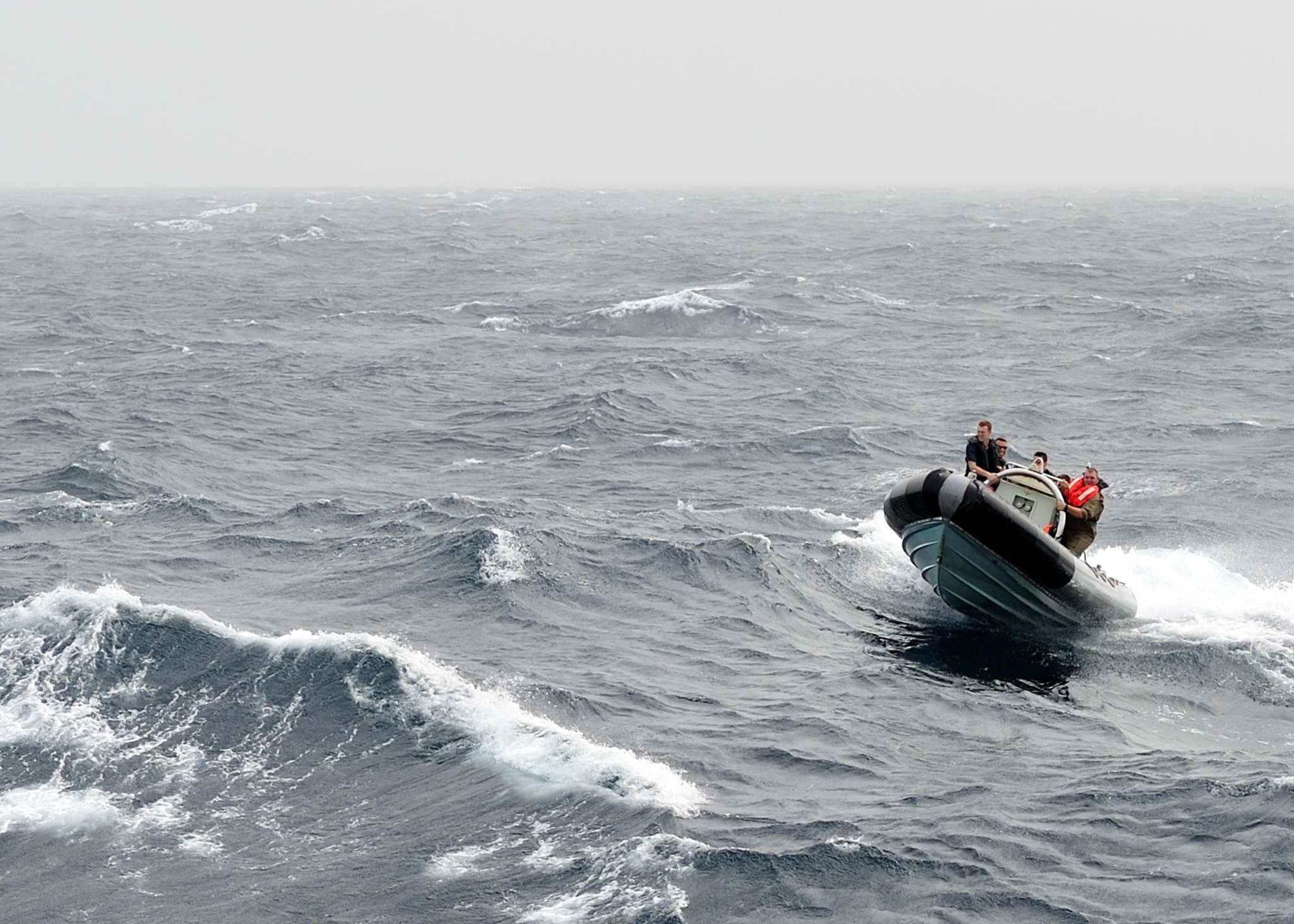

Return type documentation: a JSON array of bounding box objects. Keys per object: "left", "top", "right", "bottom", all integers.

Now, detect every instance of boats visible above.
[{"left": 883, "top": 461, "right": 1137, "bottom": 628}]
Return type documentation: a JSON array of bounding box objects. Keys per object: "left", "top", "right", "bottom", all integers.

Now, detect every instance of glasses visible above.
[
  {"left": 998, "top": 445, "right": 1008, "bottom": 450},
  {"left": 1083, "top": 475, "right": 1096, "bottom": 479}
]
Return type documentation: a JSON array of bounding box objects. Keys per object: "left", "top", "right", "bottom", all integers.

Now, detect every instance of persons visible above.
[
  {"left": 964, "top": 420, "right": 1012, "bottom": 492},
  {"left": 1034, "top": 452, "right": 1109, "bottom": 559}
]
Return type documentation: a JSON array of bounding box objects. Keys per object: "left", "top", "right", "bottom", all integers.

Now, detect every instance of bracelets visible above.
[{"left": 1064, "top": 504, "right": 1068, "bottom": 512}]
